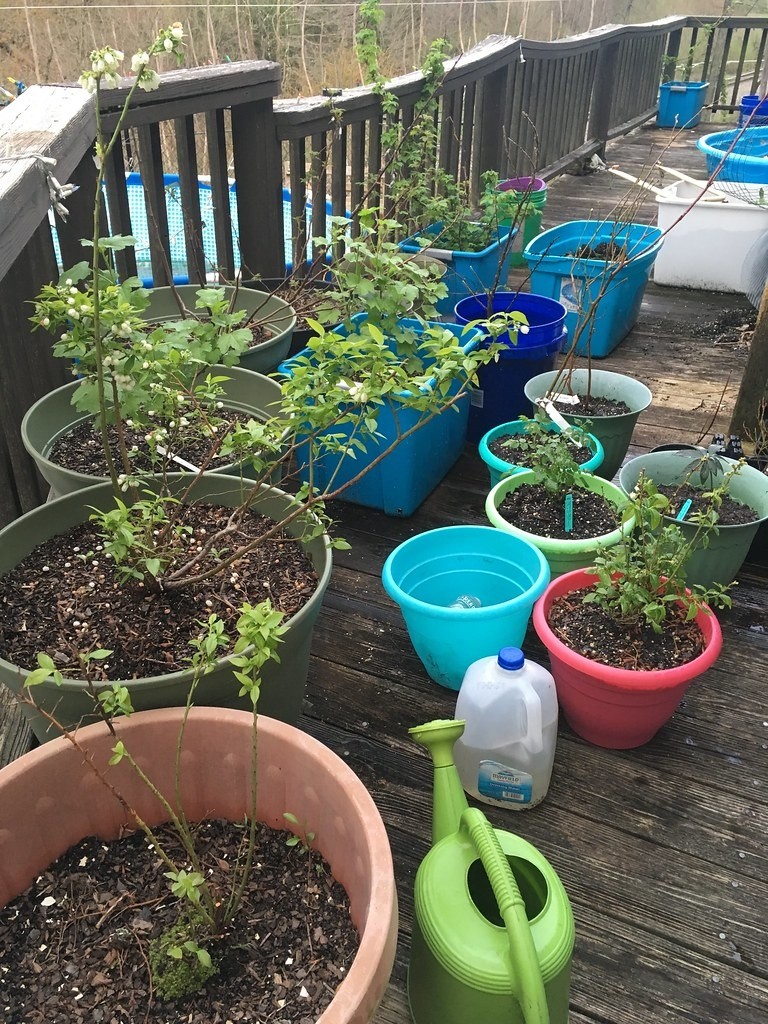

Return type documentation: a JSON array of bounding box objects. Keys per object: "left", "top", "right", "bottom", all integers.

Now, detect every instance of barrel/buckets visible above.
[
  {"left": 381, "top": 524, "right": 551, "bottom": 695},
  {"left": 737, "top": 95, "right": 768, "bottom": 129},
  {"left": 482, "top": 178, "right": 547, "bottom": 268},
  {"left": 453, "top": 289, "right": 569, "bottom": 448}
]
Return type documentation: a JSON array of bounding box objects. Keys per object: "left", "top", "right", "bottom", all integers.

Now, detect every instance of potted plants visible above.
[{"left": 0, "top": 0, "right": 767, "bottom": 1012}]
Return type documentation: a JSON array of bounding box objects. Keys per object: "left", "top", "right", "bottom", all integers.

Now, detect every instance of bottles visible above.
[
  {"left": 446, "top": 595, "right": 482, "bottom": 609},
  {"left": 451, "top": 645, "right": 558, "bottom": 812}
]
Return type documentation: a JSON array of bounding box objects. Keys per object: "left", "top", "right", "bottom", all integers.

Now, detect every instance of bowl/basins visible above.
[{"left": 696, "top": 124, "right": 768, "bottom": 184}]
